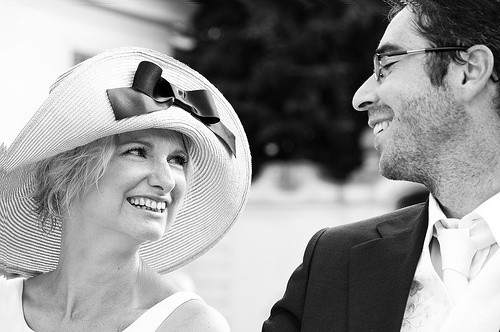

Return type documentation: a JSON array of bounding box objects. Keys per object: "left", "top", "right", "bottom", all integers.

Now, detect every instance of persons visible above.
[
  {"left": 260, "top": 1, "right": 500, "bottom": 332},
  {"left": 0, "top": 47, "right": 252, "bottom": 332}
]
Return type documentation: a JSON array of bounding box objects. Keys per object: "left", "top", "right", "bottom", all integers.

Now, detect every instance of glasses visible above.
[{"left": 372, "top": 46, "right": 500, "bottom": 82}]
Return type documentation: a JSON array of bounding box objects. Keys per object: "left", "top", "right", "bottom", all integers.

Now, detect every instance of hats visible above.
[{"left": 0, "top": 48, "right": 252, "bottom": 281}]
